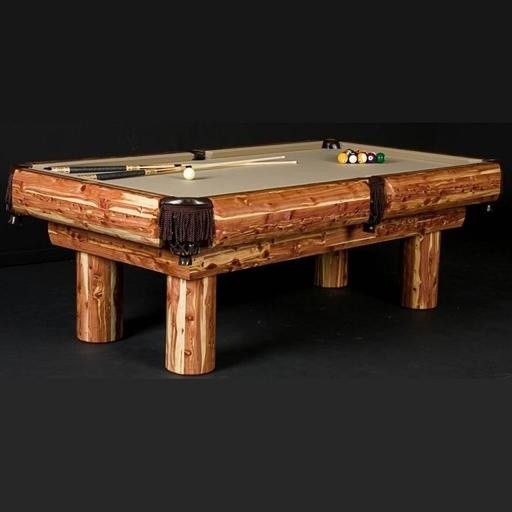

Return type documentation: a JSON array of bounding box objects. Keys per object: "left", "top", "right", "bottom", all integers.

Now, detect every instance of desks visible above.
[{"left": 6, "top": 138, "right": 501, "bottom": 377}]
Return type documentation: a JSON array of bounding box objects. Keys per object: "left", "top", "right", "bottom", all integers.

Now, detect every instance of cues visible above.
[
  {"left": 183, "top": 167, "right": 195, "bottom": 180},
  {"left": 76, "top": 155, "right": 285, "bottom": 179},
  {"left": 42, "top": 160, "right": 297, "bottom": 173}
]
